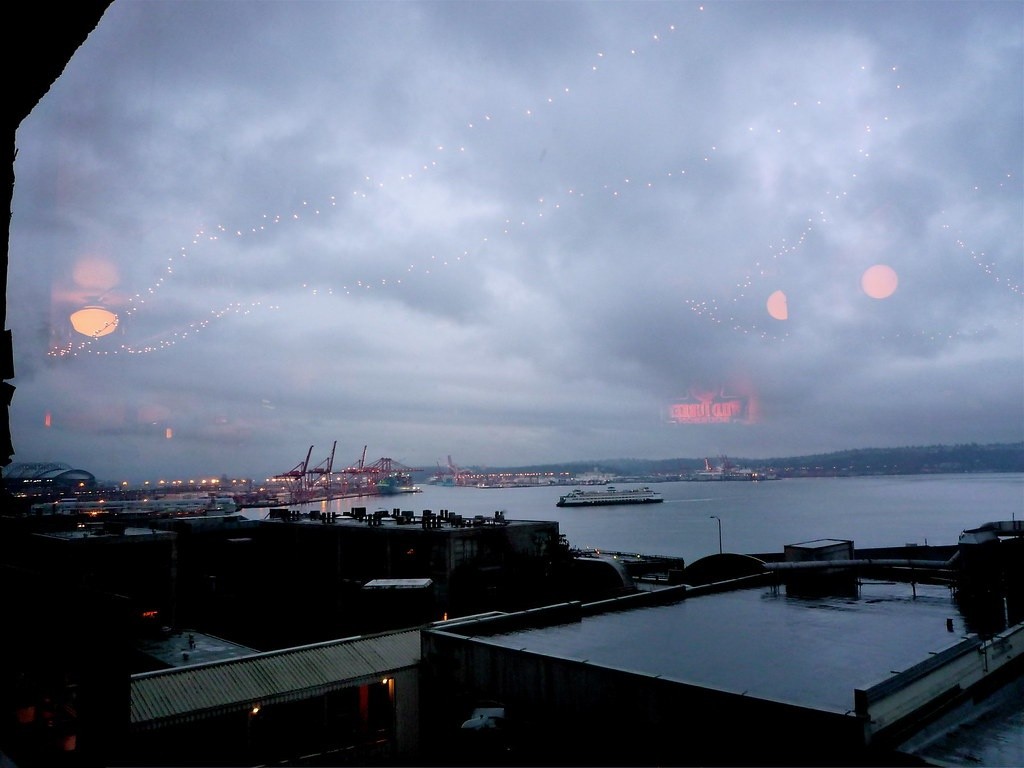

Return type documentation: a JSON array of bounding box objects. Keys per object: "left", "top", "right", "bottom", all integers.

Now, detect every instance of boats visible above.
[{"left": 555, "top": 486, "right": 665, "bottom": 506}]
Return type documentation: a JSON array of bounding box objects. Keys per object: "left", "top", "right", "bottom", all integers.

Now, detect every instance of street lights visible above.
[{"left": 710, "top": 515, "right": 722, "bottom": 555}]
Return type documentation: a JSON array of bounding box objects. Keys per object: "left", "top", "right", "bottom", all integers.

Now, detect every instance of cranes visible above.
[{"left": 285, "top": 439, "right": 424, "bottom": 504}]
